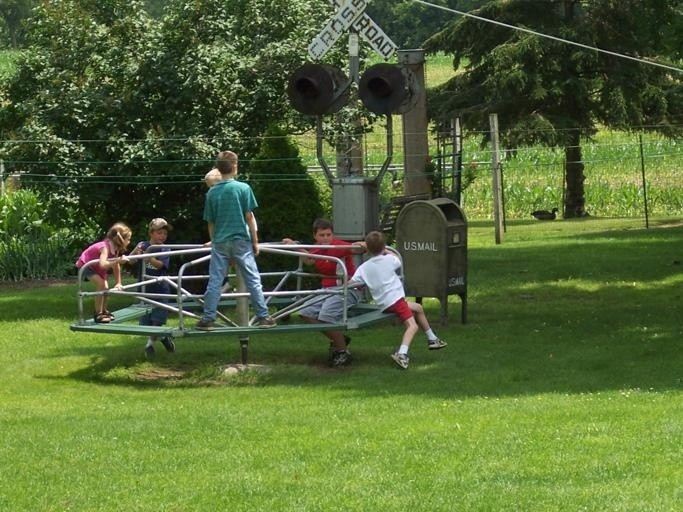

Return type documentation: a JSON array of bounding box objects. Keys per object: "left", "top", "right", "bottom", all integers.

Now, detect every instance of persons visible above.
[
  {"left": 75, "top": 220, "right": 133, "bottom": 323},
  {"left": 281, "top": 217, "right": 368, "bottom": 368},
  {"left": 202, "top": 168, "right": 259, "bottom": 293},
  {"left": 192, "top": 150, "right": 278, "bottom": 330},
  {"left": 128, "top": 217, "right": 177, "bottom": 357},
  {"left": 346, "top": 230, "right": 448, "bottom": 370}
]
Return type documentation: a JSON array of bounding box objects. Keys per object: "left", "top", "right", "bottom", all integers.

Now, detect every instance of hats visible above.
[{"left": 148, "top": 218, "right": 172, "bottom": 233}]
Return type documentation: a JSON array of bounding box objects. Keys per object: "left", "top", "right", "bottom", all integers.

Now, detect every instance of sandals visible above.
[{"left": 94, "top": 309, "right": 116, "bottom": 323}]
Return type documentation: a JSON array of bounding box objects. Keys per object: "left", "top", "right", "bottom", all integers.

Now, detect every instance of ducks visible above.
[{"left": 530, "top": 208, "right": 559, "bottom": 220}]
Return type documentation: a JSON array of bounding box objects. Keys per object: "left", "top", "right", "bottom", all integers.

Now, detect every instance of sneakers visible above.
[
  {"left": 328, "top": 334, "right": 353, "bottom": 368},
  {"left": 427, "top": 336, "right": 449, "bottom": 350},
  {"left": 257, "top": 315, "right": 277, "bottom": 329},
  {"left": 194, "top": 316, "right": 215, "bottom": 331},
  {"left": 161, "top": 335, "right": 176, "bottom": 353},
  {"left": 389, "top": 352, "right": 410, "bottom": 369},
  {"left": 144, "top": 343, "right": 156, "bottom": 361}
]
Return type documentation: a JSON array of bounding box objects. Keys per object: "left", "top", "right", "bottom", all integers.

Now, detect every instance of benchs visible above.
[{"left": 77, "top": 291, "right": 398, "bottom": 367}]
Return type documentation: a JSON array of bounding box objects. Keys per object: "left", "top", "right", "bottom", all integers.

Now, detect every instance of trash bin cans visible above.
[{"left": 396, "top": 197, "right": 468, "bottom": 326}]
